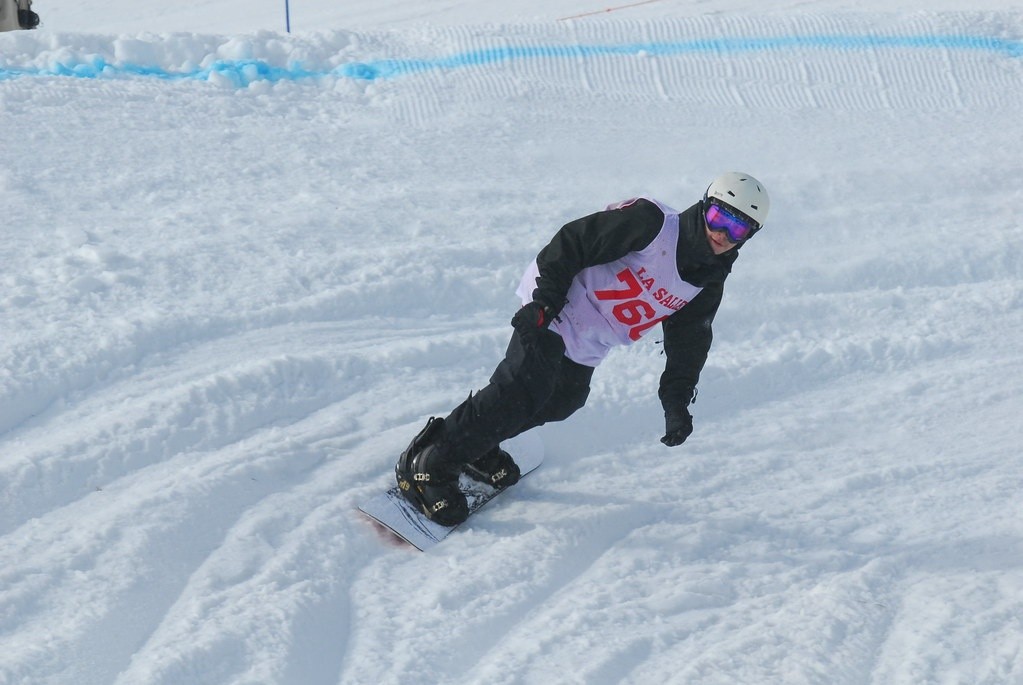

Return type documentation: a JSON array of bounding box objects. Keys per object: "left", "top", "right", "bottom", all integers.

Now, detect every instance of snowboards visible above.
[{"left": 356, "top": 427, "right": 546, "bottom": 553}]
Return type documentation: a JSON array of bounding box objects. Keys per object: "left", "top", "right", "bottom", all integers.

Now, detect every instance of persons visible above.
[{"left": 396, "top": 172, "right": 771, "bottom": 527}]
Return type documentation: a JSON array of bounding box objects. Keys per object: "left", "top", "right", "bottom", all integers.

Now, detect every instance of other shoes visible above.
[
  {"left": 409, "top": 451, "right": 469, "bottom": 525},
  {"left": 464, "top": 448, "right": 519, "bottom": 487}
]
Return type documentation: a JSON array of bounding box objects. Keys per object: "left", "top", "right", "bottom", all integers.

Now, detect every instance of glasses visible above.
[{"left": 704, "top": 204, "right": 752, "bottom": 243}]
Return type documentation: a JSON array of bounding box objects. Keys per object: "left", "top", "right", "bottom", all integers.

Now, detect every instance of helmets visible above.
[{"left": 703, "top": 170, "right": 769, "bottom": 238}]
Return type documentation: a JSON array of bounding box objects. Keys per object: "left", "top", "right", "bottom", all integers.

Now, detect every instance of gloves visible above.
[
  {"left": 658, "top": 382, "right": 693, "bottom": 447},
  {"left": 511, "top": 300, "right": 564, "bottom": 345}
]
